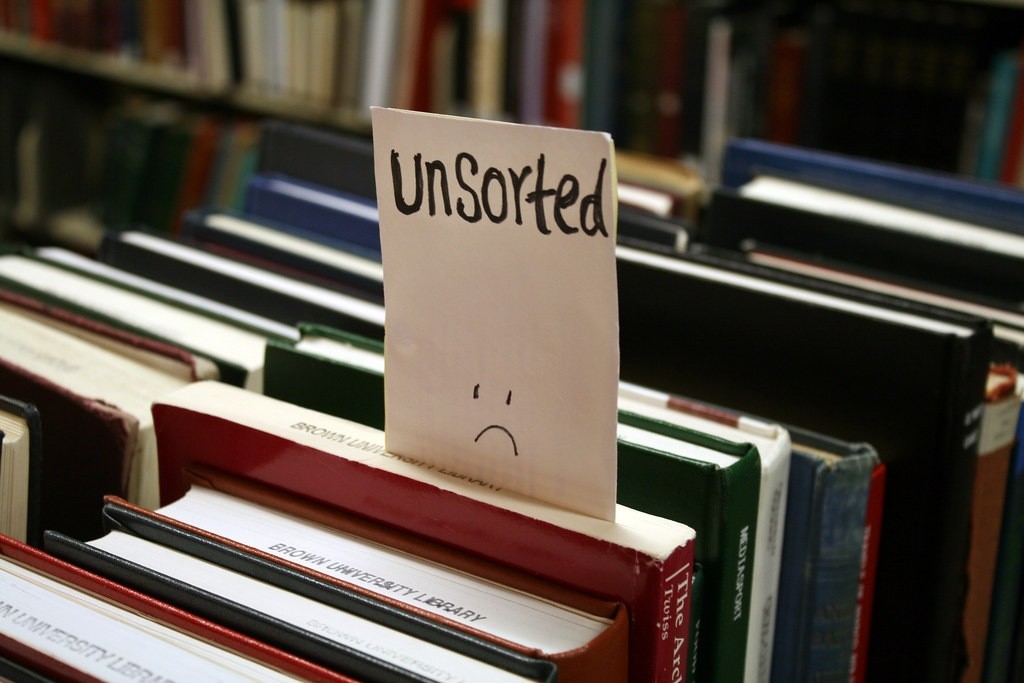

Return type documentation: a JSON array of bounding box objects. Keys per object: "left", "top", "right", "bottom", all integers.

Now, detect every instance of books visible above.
[{"left": 2, "top": 2, "right": 1023, "bottom": 683}]
